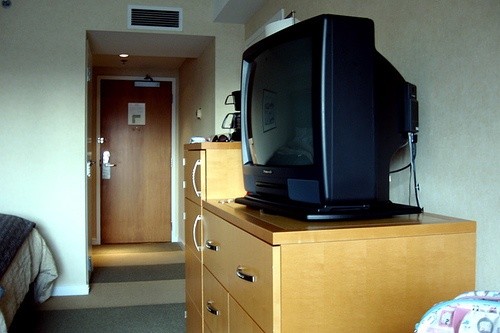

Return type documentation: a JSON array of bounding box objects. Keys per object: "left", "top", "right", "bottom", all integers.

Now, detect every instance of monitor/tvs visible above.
[{"left": 232, "top": 13, "right": 424, "bottom": 223}]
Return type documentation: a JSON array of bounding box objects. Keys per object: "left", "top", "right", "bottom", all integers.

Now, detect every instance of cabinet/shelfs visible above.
[
  {"left": 201, "top": 204, "right": 476, "bottom": 333},
  {"left": 181, "top": 143, "right": 245, "bottom": 332}
]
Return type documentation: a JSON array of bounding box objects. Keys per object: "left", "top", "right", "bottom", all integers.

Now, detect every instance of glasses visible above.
[{"left": 212, "top": 135, "right": 232, "bottom": 142}]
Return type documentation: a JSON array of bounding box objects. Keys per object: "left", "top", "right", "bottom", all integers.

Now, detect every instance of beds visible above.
[{"left": 0, "top": 213, "right": 57, "bottom": 333}]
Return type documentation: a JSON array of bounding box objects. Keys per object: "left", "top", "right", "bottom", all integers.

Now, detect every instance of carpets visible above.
[
  {"left": 8, "top": 304, "right": 185, "bottom": 333},
  {"left": 90, "top": 263, "right": 185, "bottom": 282}
]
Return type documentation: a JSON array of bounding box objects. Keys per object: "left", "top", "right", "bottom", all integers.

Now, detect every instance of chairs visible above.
[{"left": 415, "top": 290, "right": 500, "bottom": 332}]
